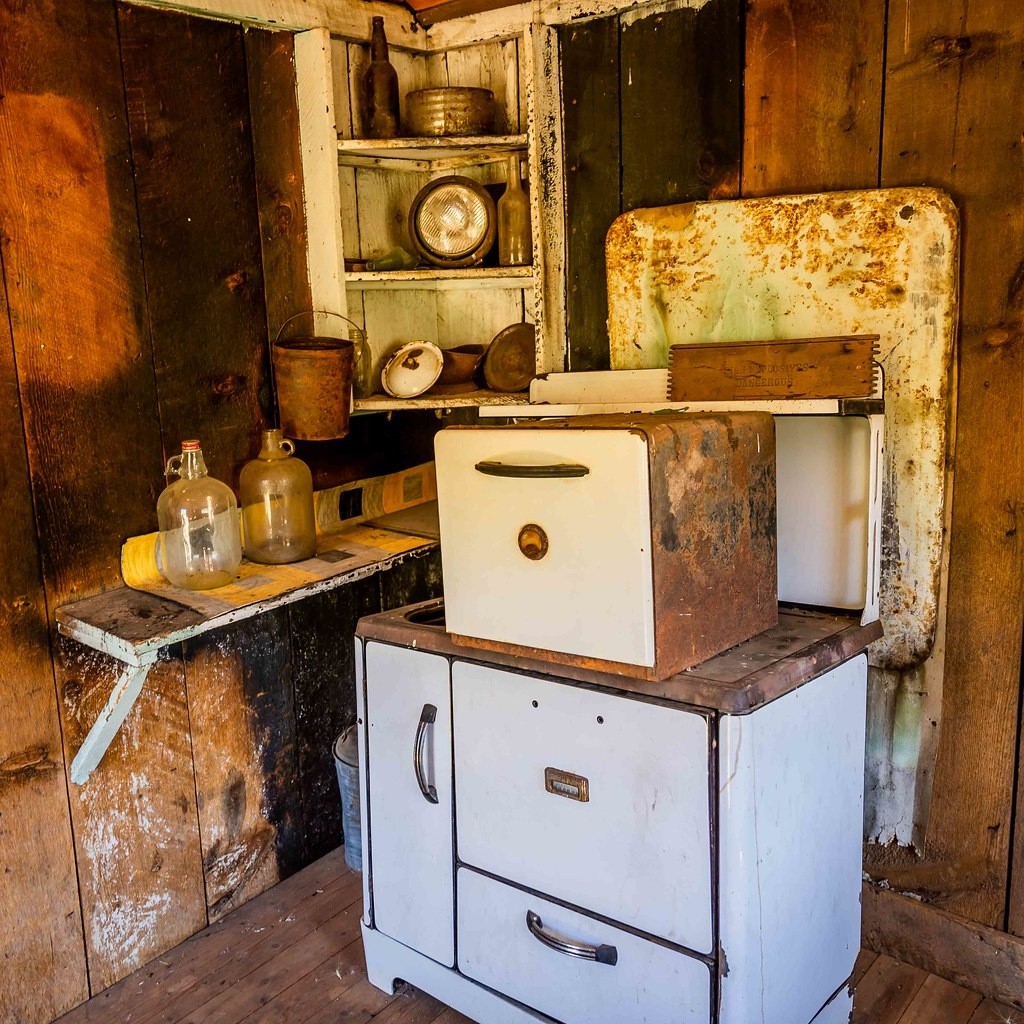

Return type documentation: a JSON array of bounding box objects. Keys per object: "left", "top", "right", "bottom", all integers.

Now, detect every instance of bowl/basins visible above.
[{"left": 436, "top": 344, "right": 484, "bottom": 386}]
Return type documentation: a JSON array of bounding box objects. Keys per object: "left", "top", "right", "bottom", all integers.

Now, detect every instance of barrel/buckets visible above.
[{"left": 333, "top": 725, "right": 370, "bottom": 873}]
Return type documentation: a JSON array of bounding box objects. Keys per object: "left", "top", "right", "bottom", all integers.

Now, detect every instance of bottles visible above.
[
  {"left": 496, "top": 151, "right": 533, "bottom": 269},
  {"left": 154, "top": 440, "right": 242, "bottom": 593},
  {"left": 239, "top": 426, "right": 318, "bottom": 568},
  {"left": 358, "top": 15, "right": 402, "bottom": 138},
  {"left": 345, "top": 329, "right": 374, "bottom": 397}
]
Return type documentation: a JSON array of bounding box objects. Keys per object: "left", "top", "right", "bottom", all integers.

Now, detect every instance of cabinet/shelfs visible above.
[
  {"left": 336, "top": 133, "right": 539, "bottom": 411},
  {"left": 54, "top": 496, "right": 442, "bottom": 785},
  {"left": 353, "top": 334, "right": 892, "bottom": 1024}
]
